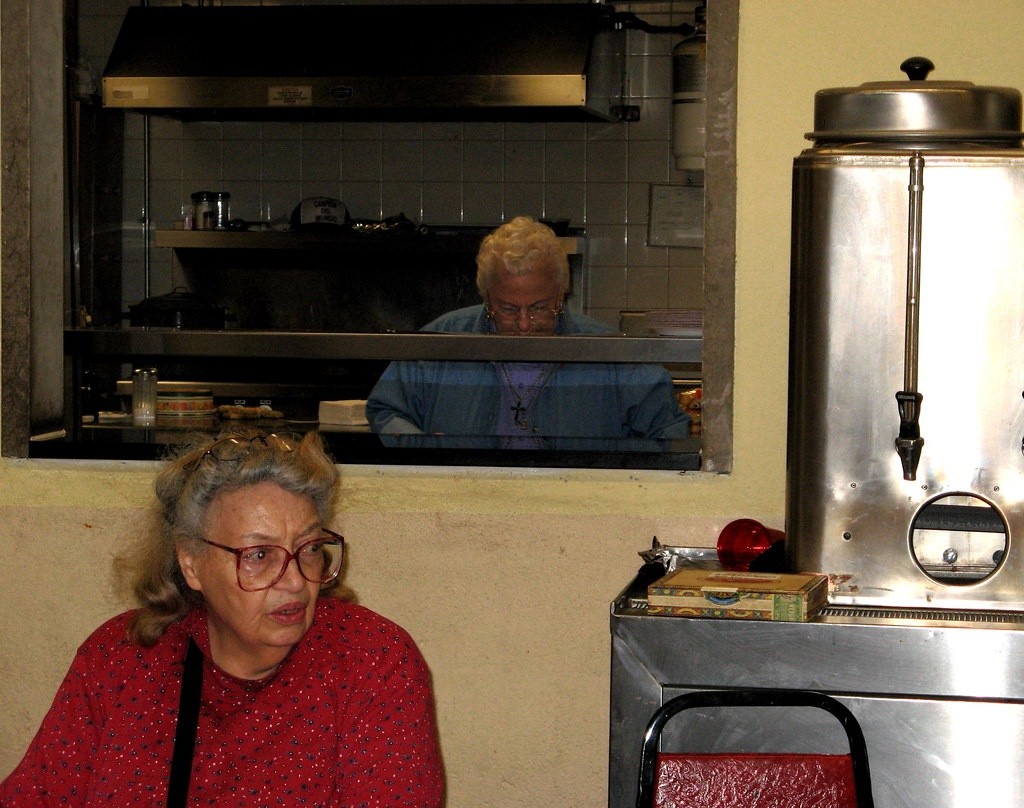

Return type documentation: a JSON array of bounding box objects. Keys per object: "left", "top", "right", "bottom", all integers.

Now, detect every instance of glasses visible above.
[
  {"left": 190, "top": 528, "right": 345, "bottom": 592},
  {"left": 485, "top": 286, "right": 560, "bottom": 323},
  {"left": 195, "top": 433, "right": 299, "bottom": 471}
]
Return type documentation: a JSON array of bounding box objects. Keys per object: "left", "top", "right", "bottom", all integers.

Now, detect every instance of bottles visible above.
[{"left": 189, "top": 191, "right": 231, "bottom": 232}]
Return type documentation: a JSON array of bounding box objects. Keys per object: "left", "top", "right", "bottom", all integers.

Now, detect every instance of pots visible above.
[{"left": 126, "top": 286, "right": 224, "bottom": 383}]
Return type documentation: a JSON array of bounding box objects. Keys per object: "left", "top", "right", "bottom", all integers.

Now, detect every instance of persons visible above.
[
  {"left": 363, "top": 214, "right": 694, "bottom": 439},
  {"left": 0, "top": 428, "right": 448, "bottom": 808}
]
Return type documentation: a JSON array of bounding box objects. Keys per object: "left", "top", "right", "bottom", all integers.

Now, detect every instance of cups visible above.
[
  {"left": 715, "top": 518, "right": 785, "bottom": 572},
  {"left": 130, "top": 367, "right": 157, "bottom": 428}
]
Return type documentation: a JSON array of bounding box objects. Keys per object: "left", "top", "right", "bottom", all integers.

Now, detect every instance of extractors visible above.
[{"left": 98, "top": 0, "right": 644, "bottom": 127}]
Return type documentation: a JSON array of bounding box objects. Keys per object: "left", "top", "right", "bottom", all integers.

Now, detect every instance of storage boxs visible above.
[{"left": 648, "top": 569, "right": 828, "bottom": 624}]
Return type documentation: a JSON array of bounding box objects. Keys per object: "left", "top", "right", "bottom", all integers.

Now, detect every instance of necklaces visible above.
[{"left": 500, "top": 362, "right": 548, "bottom": 432}]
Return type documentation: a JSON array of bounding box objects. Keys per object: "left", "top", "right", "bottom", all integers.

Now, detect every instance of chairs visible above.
[{"left": 634, "top": 691, "right": 874, "bottom": 807}]
